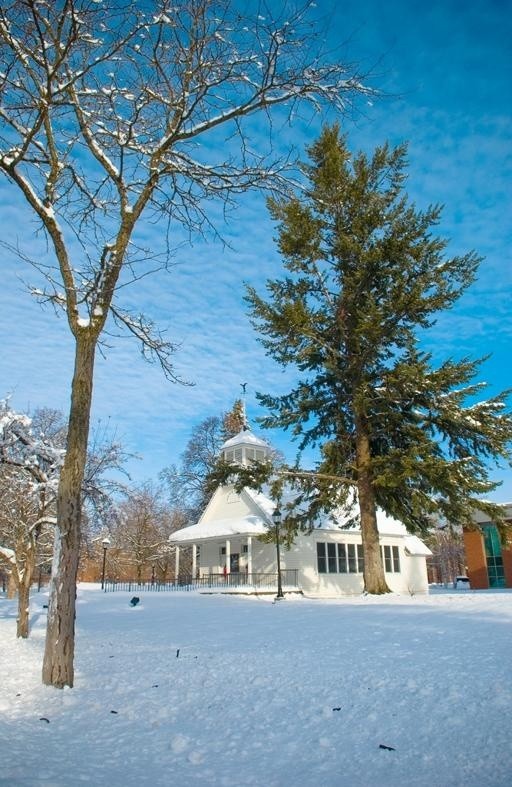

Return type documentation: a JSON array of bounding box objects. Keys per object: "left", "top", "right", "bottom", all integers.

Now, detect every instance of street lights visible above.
[
  {"left": 271, "top": 507, "right": 286, "bottom": 601},
  {"left": 101, "top": 538, "right": 110, "bottom": 589}
]
